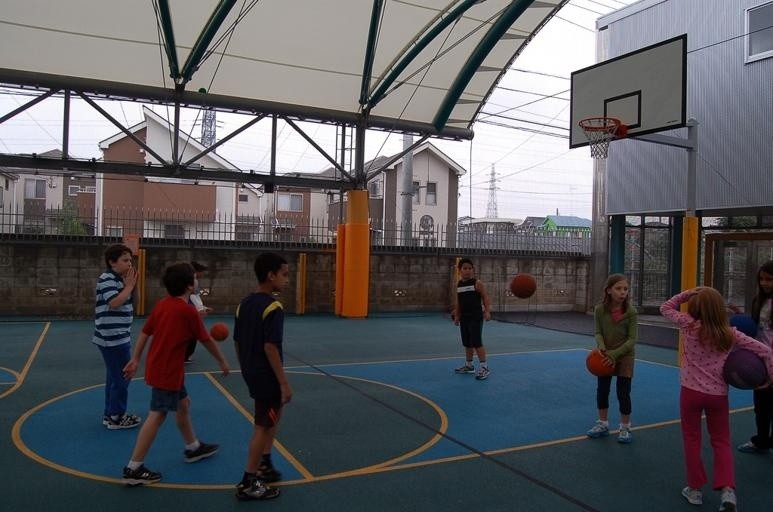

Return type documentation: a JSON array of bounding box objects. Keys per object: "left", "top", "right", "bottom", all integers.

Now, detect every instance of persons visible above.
[
  {"left": 656, "top": 283, "right": 773, "bottom": 511},
  {"left": 89, "top": 243, "right": 142, "bottom": 430},
  {"left": 182, "top": 260, "right": 213, "bottom": 366},
  {"left": 450, "top": 256, "right": 492, "bottom": 382},
  {"left": 118, "top": 259, "right": 231, "bottom": 484},
  {"left": 585, "top": 268, "right": 638, "bottom": 443},
  {"left": 230, "top": 251, "right": 293, "bottom": 504},
  {"left": 725, "top": 256, "right": 773, "bottom": 458}
]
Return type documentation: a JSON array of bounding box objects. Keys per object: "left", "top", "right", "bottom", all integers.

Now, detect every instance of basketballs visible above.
[
  {"left": 508, "top": 273, "right": 537, "bottom": 299},
  {"left": 209, "top": 323, "right": 229, "bottom": 341},
  {"left": 722, "top": 349, "right": 767, "bottom": 390},
  {"left": 727, "top": 313, "right": 757, "bottom": 340},
  {"left": 586, "top": 349, "right": 616, "bottom": 376}
]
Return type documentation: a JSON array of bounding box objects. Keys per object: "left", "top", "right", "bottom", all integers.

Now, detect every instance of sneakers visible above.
[
  {"left": 183, "top": 440, "right": 221, "bottom": 463},
  {"left": 102, "top": 411, "right": 143, "bottom": 430},
  {"left": 474, "top": 364, "right": 492, "bottom": 381},
  {"left": 235, "top": 477, "right": 281, "bottom": 501},
  {"left": 617, "top": 421, "right": 634, "bottom": 443},
  {"left": 120, "top": 463, "right": 163, "bottom": 486},
  {"left": 183, "top": 358, "right": 193, "bottom": 364},
  {"left": 255, "top": 465, "right": 282, "bottom": 484},
  {"left": 682, "top": 485, "right": 704, "bottom": 506},
  {"left": 717, "top": 486, "right": 737, "bottom": 512},
  {"left": 736, "top": 442, "right": 770, "bottom": 453},
  {"left": 454, "top": 362, "right": 476, "bottom": 375},
  {"left": 586, "top": 419, "right": 611, "bottom": 438}
]
